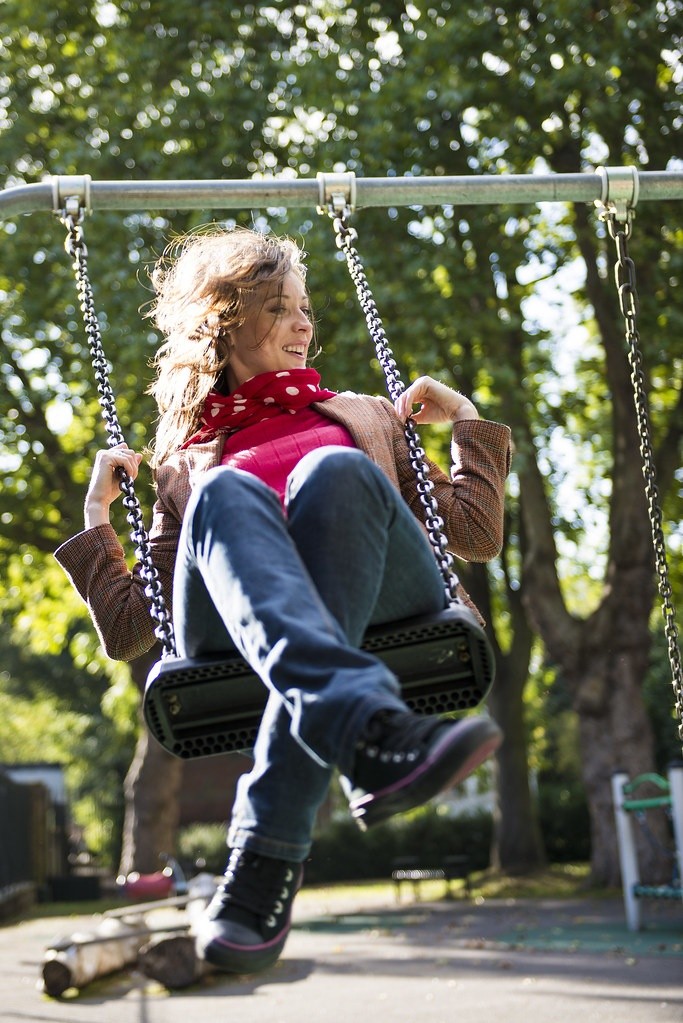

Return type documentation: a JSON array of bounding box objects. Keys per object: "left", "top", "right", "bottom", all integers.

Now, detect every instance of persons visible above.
[{"left": 55, "top": 234, "right": 515, "bottom": 972}]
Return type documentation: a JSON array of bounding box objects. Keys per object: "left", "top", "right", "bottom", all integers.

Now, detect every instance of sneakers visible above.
[
  {"left": 337, "top": 707, "right": 502, "bottom": 830},
  {"left": 194, "top": 847, "right": 303, "bottom": 971}
]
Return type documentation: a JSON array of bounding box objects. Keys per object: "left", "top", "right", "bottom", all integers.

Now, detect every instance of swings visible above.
[
  {"left": 601, "top": 217, "right": 683, "bottom": 738},
  {"left": 63, "top": 212, "right": 496, "bottom": 758}
]
